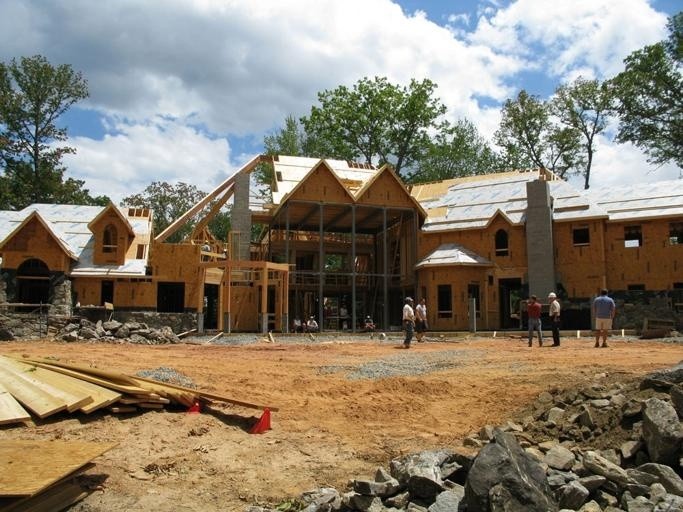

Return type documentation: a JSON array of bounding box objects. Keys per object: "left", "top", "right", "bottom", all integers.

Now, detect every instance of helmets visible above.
[{"left": 548, "top": 293, "right": 557, "bottom": 298}]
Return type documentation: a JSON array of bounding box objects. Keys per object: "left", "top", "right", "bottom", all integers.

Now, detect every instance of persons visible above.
[
  {"left": 548, "top": 292, "right": 560, "bottom": 346},
  {"left": 592, "top": 288, "right": 615, "bottom": 347},
  {"left": 528, "top": 295, "right": 543, "bottom": 347},
  {"left": 340, "top": 305, "right": 348, "bottom": 330},
  {"left": 364, "top": 316, "right": 376, "bottom": 332},
  {"left": 293, "top": 315, "right": 319, "bottom": 333},
  {"left": 414, "top": 296, "right": 428, "bottom": 343},
  {"left": 400, "top": 297, "right": 415, "bottom": 348}
]
